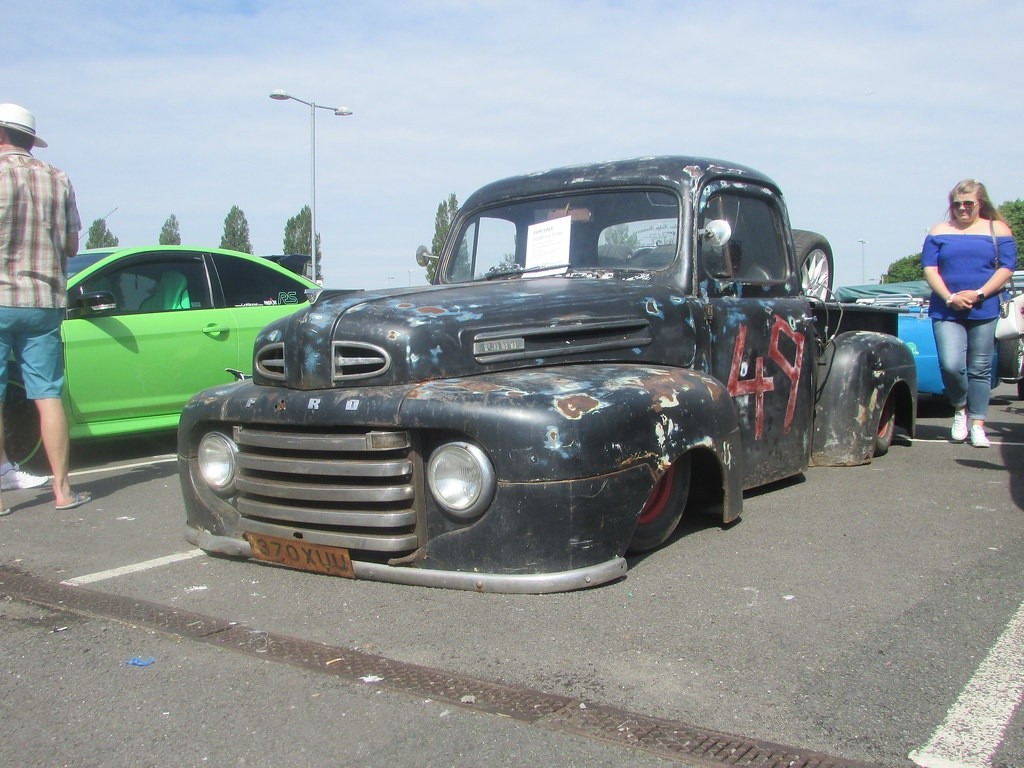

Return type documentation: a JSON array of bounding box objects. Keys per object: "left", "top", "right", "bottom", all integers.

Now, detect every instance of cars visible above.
[{"left": 0, "top": 246, "right": 323, "bottom": 473}]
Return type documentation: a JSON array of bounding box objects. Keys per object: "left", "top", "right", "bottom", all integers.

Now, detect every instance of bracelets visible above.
[{"left": 946, "top": 293, "right": 956, "bottom": 308}]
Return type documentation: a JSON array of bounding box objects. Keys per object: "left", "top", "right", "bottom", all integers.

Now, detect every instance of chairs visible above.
[
  {"left": 244, "top": 268, "right": 284, "bottom": 305},
  {"left": 138, "top": 270, "right": 191, "bottom": 313}
]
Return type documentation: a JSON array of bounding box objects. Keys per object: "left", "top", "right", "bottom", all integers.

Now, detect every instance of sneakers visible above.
[
  {"left": 0, "top": 462, "right": 49, "bottom": 490},
  {"left": 951, "top": 411, "right": 968, "bottom": 440},
  {"left": 971, "top": 424, "right": 990, "bottom": 448}
]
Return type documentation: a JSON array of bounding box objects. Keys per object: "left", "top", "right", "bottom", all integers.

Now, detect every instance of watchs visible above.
[{"left": 976, "top": 289, "right": 985, "bottom": 303}]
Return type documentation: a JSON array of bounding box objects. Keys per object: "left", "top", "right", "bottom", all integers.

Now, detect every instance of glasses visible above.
[{"left": 952, "top": 201, "right": 979, "bottom": 209}]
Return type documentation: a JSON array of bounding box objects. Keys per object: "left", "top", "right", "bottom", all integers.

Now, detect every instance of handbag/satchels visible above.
[{"left": 994, "top": 294, "right": 1024, "bottom": 340}]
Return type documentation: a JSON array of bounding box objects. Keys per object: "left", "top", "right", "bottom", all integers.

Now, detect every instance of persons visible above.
[
  {"left": 921, "top": 178, "right": 1017, "bottom": 448},
  {"left": 1, "top": 102, "right": 94, "bottom": 510}
]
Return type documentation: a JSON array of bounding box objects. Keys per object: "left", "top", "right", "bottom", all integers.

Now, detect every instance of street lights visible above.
[
  {"left": 269, "top": 89, "right": 354, "bottom": 286},
  {"left": 857, "top": 240, "right": 866, "bottom": 285}
]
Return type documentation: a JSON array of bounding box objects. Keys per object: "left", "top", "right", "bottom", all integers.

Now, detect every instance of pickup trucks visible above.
[{"left": 178, "top": 155, "right": 917, "bottom": 595}]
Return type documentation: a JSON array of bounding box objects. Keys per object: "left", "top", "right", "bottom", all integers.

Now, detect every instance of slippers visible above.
[
  {"left": 0, "top": 508, "right": 10, "bottom": 516},
  {"left": 56, "top": 492, "right": 91, "bottom": 509}
]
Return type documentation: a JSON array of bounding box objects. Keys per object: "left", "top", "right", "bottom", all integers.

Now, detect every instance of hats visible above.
[{"left": 0, "top": 103, "right": 48, "bottom": 148}]
